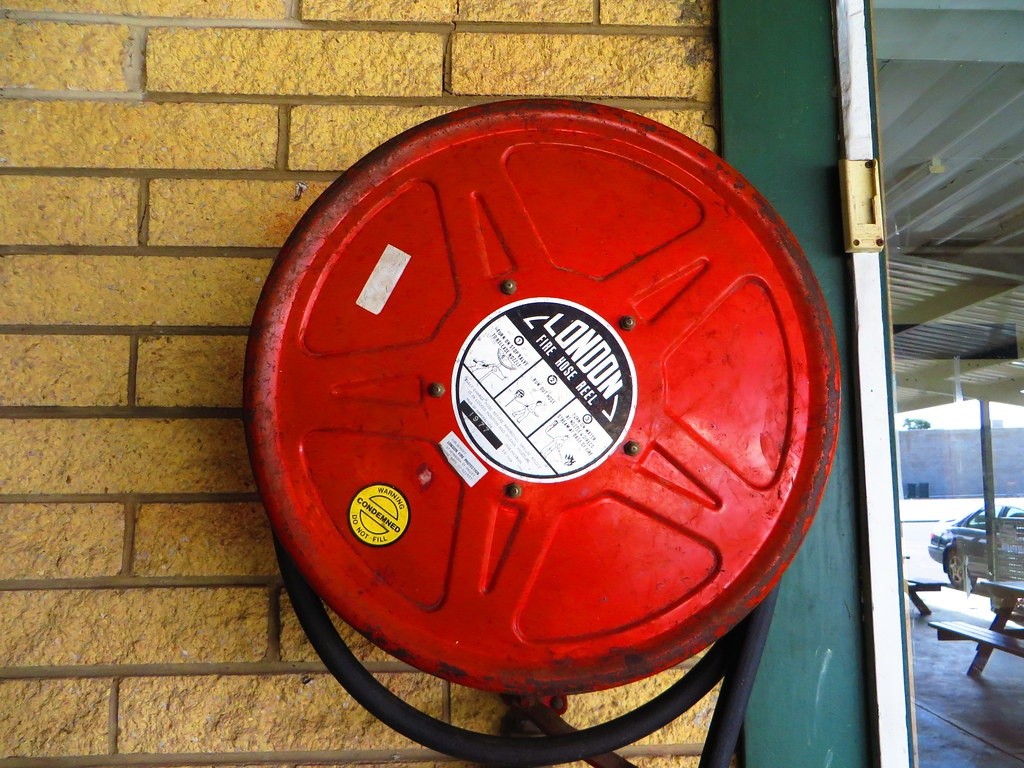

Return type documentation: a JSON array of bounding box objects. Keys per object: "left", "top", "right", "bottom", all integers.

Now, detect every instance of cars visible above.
[{"left": 927, "top": 503, "right": 1024, "bottom": 592}]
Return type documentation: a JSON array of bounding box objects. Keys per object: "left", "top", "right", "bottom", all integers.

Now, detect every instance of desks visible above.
[{"left": 965, "top": 581, "right": 1024, "bottom": 676}]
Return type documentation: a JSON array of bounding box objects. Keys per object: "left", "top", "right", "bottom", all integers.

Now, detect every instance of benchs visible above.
[
  {"left": 907, "top": 579, "right": 947, "bottom": 591},
  {"left": 928, "top": 621, "right": 1024, "bottom": 675}
]
